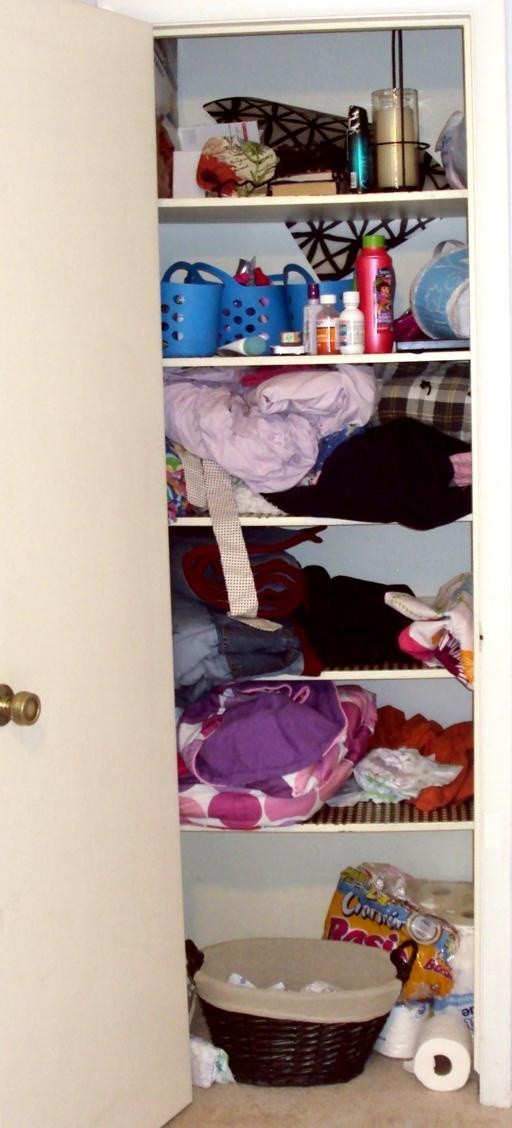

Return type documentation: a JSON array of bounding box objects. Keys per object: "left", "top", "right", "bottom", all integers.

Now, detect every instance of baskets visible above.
[
  {"left": 184, "top": 937, "right": 420, "bottom": 1090},
  {"left": 160, "top": 259, "right": 355, "bottom": 360}
]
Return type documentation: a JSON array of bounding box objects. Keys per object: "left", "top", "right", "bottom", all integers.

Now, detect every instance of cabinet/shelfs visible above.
[{"left": 0, "top": 18, "right": 481, "bottom": 1128}]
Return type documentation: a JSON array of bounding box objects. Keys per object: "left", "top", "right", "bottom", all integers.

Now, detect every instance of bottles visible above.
[
  {"left": 305, "top": 234, "right": 397, "bottom": 355},
  {"left": 345, "top": 103, "right": 376, "bottom": 193}
]
